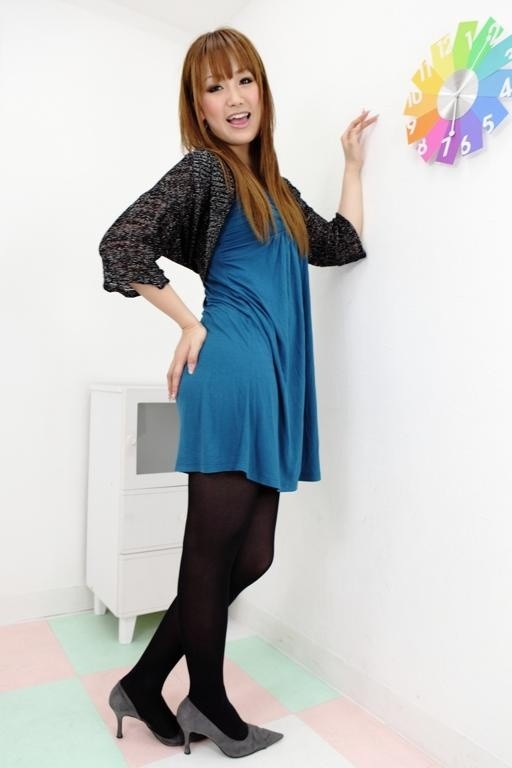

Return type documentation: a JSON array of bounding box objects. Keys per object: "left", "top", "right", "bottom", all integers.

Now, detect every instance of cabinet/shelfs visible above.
[{"left": 86, "top": 386, "right": 190, "bottom": 645}]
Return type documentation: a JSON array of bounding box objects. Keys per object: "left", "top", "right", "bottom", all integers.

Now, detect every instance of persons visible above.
[{"left": 97, "top": 27, "right": 381, "bottom": 758}]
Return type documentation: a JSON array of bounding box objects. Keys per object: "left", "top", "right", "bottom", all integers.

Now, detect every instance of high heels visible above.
[
  {"left": 177, "top": 695, "right": 283, "bottom": 758},
  {"left": 109, "top": 681, "right": 207, "bottom": 746}
]
